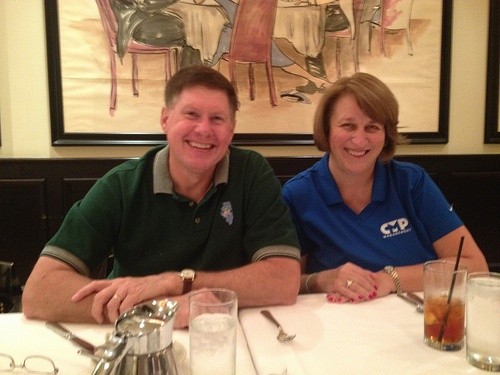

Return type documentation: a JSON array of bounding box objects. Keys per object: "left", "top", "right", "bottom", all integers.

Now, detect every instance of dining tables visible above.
[
  {"left": 0, "top": 307, "right": 258, "bottom": 375},
  {"left": 237, "top": 275, "right": 500, "bottom": 375}
]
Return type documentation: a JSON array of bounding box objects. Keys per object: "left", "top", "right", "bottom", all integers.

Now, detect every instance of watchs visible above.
[
  {"left": 384, "top": 265, "right": 402, "bottom": 292},
  {"left": 180, "top": 268, "right": 196, "bottom": 294}
]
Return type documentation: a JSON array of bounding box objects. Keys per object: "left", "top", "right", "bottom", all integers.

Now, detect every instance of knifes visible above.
[{"left": 46, "top": 320, "right": 96, "bottom": 353}]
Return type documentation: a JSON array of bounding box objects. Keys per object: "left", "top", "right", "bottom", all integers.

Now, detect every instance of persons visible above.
[
  {"left": 22, "top": 60, "right": 301, "bottom": 325},
  {"left": 279, "top": 72, "right": 490, "bottom": 304}
]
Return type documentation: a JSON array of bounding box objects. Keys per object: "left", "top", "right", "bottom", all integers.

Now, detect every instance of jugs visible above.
[{"left": 91, "top": 298, "right": 181, "bottom": 375}]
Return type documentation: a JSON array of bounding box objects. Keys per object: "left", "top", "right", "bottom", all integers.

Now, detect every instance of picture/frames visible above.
[{"left": 44, "top": 0, "right": 452, "bottom": 146}]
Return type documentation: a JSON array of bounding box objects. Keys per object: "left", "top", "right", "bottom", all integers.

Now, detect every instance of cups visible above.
[
  {"left": 424, "top": 258, "right": 470, "bottom": 351},
  {"left": 466, "top": 271, "right": 499, "bottom": 372},
  {"left": 188, "top": 289, "right": 238, "bottom": 375}
]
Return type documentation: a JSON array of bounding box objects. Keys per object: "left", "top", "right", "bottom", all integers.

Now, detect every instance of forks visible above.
[{"left": 260, "top": 310, "right": 297, "bottom": 341}]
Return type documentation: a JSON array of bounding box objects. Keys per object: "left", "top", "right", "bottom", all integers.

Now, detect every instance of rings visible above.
[
  {"left": 347, "top": 280, "right": 353, "bottom": 287},
  {"left": 114, "top": 295, "right": 122, "bottom": 300}
]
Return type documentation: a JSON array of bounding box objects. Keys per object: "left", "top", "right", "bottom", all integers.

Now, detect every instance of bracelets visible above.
[{"left": 305, "top": 273, "right": 314, "bottom": 293}]
